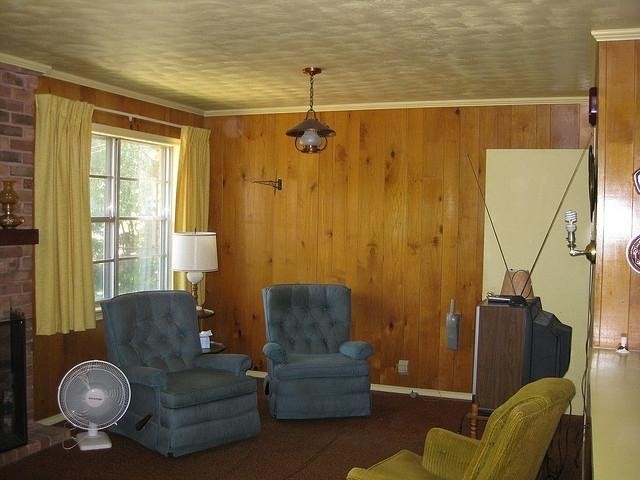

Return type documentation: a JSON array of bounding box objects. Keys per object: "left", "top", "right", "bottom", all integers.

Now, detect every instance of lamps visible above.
[
  {"left": 171, "top": 227, "right": 219, "bottom": 315},
  {"left": 564, "top": 210, "right": 596, "bottom": 264},
  {"left": 285, "top": 67, "right": 336, "bottom": 154}
]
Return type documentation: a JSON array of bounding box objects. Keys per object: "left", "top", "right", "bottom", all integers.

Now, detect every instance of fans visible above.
[{"left": 58, "top": 360, "right": 131, "bottom": 451}]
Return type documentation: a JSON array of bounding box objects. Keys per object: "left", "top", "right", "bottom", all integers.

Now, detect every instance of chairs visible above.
[
  {"left": 262, "top": 284, "right": 374, "bottom": 421},
  {"left": 100, "top": 290, "right": 261, "bottom": 457},
  {"left": 346, "top": 377, "right": 576, "bottom": 480}
]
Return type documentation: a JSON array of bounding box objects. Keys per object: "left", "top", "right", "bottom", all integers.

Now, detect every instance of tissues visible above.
[{"left": 199, "top": 329, "right": 213, "bottom": 349}]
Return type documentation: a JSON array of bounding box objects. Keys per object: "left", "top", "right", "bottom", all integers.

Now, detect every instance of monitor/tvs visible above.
[{"left": 473, "top": 297, "right": 573, "bottom": 415}]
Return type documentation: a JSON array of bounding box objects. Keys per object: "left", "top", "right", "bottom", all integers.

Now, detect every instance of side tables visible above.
[
  {"left": 466, "top": 402, "right": 574, "bottom": 439},
  {"left": 197, "top": 309, "right": 226, "bottom": 354}
]
